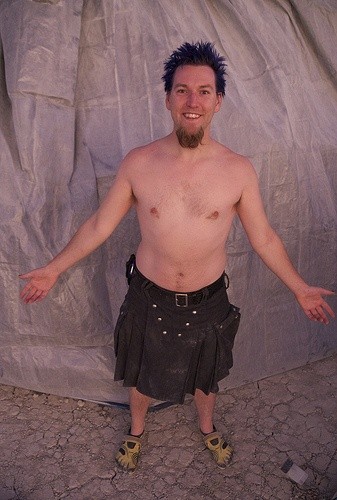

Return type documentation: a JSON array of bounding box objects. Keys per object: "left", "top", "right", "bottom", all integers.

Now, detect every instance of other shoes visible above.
[
  {"left": 115, "top": 425, "right": 144, "bottom": 471},
  {"left": 199, "top": 426, "right": 233, "bottom": 468}
]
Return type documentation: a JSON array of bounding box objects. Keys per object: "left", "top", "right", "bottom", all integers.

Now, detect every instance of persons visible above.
[{"left": 19, "top": 40, "right": 335, "bottom": 471}]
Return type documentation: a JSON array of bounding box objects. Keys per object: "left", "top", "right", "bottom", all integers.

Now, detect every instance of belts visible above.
[{"left": 130, "top": 268, "right": 225, "bottom": 306}]
